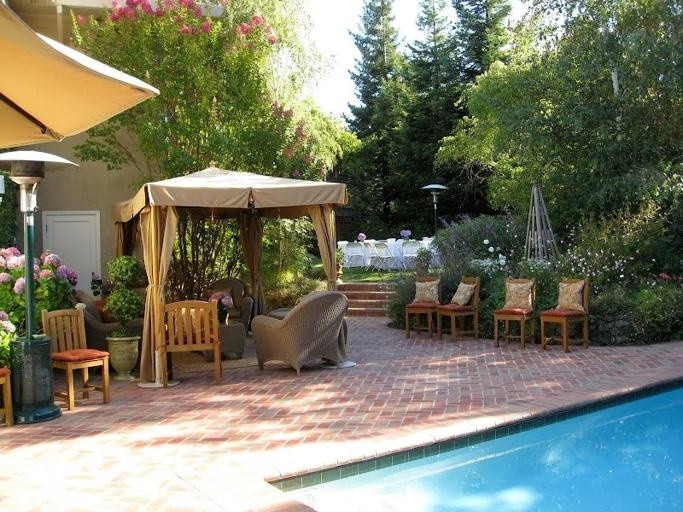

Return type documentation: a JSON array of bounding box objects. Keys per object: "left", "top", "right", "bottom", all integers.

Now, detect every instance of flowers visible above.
[
  {"left": 208, "top": 292, "right": 234, "bottom": 310},
  {"left": 400, "top": 230, "right": 411, "bottom": 236},
  {"left": 357, "top": 232, "right": 366, "bottom": 240}
]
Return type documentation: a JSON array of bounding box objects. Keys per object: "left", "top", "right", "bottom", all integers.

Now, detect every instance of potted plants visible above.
[{"left": 103, "top": 289, "right": 144, "bottom": 382}]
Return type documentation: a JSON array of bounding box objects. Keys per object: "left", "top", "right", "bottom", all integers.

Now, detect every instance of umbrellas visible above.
[{"left": 0, "top": 0, "right": 160, "bottom": 148}]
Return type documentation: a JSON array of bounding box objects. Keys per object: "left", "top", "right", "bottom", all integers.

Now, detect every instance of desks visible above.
[{"left": 196, "top": 319, "right": 246, "bottom": 361}]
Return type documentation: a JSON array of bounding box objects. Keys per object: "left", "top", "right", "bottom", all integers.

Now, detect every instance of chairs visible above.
[
  {"left": 41, "top": 307, "right": 112, "bottom": 410},
  {"left": 493, "top": 277, "right": 536, "bottom": 349},
  {"left": 336, "top": 236, "right": 441, "bottom": 272},
  {"left": 405, "top": 275, "right": 442, "bottom": 338},
  {"left": 160, "top": 299, "right": 223, "bottom": 386},
  {"left": 66, "top": 290, "right": 145, "bottom": 373},
  {"left": 251, "top": 290, "right": 350, "bottom": 376},
  {"left": 540, "top": 277, "right": 590, "bottom": 354},
  {"left": 0, "top": 368, "right": 15, "bottom": 427},
  {"left": 437, "top": 276, "right": 481, "bottom": 340},
  {"left": 201, "top": 277, "right": 252, "bottom": 336}
]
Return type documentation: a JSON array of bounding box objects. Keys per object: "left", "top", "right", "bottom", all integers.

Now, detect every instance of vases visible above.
[{"left": 218, "top": 306, "right": 230, "bottom": 326}]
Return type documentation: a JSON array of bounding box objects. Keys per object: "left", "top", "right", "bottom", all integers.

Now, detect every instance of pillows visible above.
[
  {"left": 411, "top": 278, "right": 440, "bottom": 305},
  {"left": 450, "top": 281, "right": 475, "bottom": 306},
  {"left": 503, "top": 282, "right": 533, "bottom": 310},
  {"left": 206, "top": 287, "right": 232, "bottom": 302},
  {"left": 555, "top": 280, "right": 585, "bottom": 312}
]
